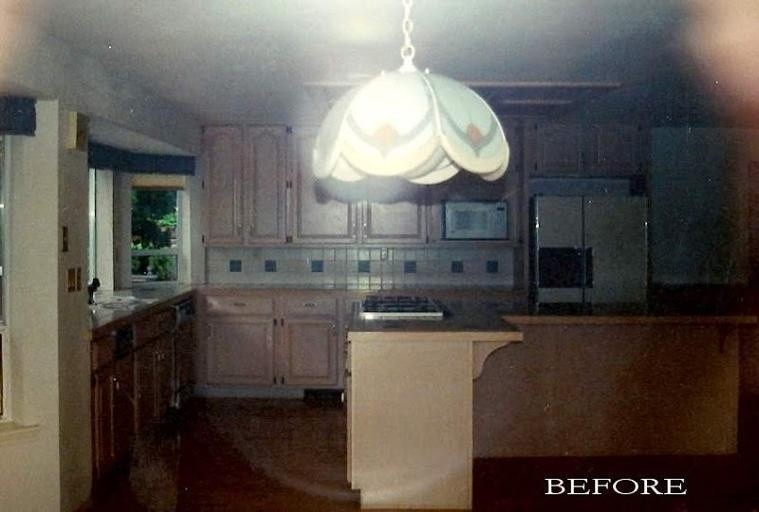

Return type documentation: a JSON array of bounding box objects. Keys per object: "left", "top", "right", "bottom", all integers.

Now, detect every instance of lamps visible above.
[{"left": 311, "top": 0, "right": 510, "bottom": 186}]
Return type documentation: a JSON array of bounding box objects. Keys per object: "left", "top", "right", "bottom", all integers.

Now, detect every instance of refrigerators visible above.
[{"left": 529, "top": 192, "right": 650, "bottom": 314}]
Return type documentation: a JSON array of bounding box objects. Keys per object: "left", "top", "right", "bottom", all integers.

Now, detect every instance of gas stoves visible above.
[{"left": 359, "top": 294, "right": 445, "bottom": 318}]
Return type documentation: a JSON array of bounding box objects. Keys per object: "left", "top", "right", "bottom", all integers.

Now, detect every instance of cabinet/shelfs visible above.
[
  {"left": 209, "top": 124, "right": 429, "bottom": 245},
  {"left": 94, "top": 297, "right": 194, "bottom": 479},
  {"left": 524, "top": 119, "right": 649, "bottom": 178},
  {"left": 204, "top": 297, "right": 337, "bottom": 388}
]
